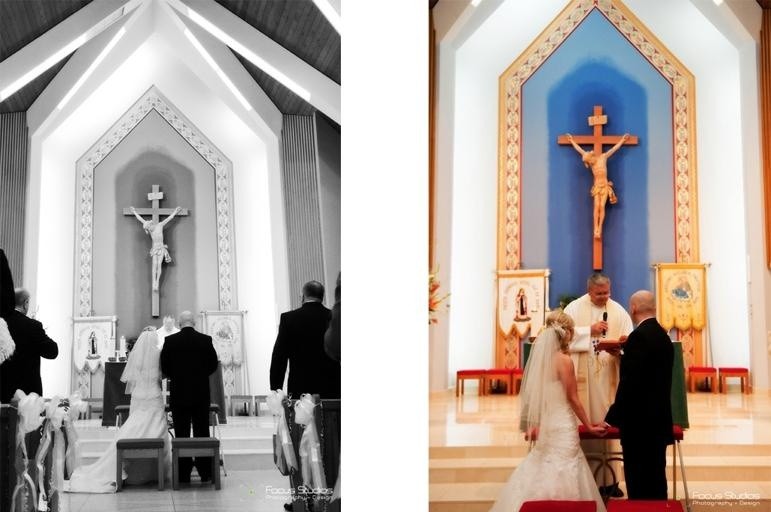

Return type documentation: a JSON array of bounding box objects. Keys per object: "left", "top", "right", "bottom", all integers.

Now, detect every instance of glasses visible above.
[{"left": 592, "top": 291, "right": 611, "bottom": 299}]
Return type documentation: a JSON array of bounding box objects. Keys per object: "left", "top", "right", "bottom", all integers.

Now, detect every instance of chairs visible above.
[
  {"left": 295, "top": 393, "right": 341, "bottom": 511},
  {"left": 22, "top": 415, "right": 59, "bottom": 512},
  {"left": 0, "top": 398, "right": 37, "bottom": 512},
  {"left": 268, "top": 389, "right": 304, "bottom": 497},
  {"left": 41, "top": 398, "right": 76, "bottom": 479}
]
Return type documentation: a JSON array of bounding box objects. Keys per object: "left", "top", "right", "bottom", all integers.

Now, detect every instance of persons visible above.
[
  {"left": 559, "top": 271, "right": 634, "bottom": 497},
  {"left": 269, "top": 280, "right": 335, "bottom": 511},
  {"left": 487, "top": 311, "right": 607, "bottom": 512},
  {"left": 130, "top": 205, "right": 182, "bottom": 292},
  {"left": 156, "top": 316, "right": 181, "bottom": 352},
  {"left": 87, "top": 331, "right": 97, "bottom": 354},
  {"left": 567, "top": 132, "right": 630, "bottom": 241},
  {"left": 62, "top": 325, "right": 167, "bottom": 494},
  {"left": 515, "top": 288, "right": 528, "bottom": 316},
  {"left": 11, "top": 288, "right": 59, "bottom": 459},
  {"left": 597, "top": 290, "right": 675, "bottom": 502},
  {"left": 159, "top": 310, "right": 218, "bottom": 484}
]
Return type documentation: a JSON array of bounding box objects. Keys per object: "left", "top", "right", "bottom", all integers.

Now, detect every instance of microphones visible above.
[{"left": 603, "top": 312, "right": 607, "bottom": 339}]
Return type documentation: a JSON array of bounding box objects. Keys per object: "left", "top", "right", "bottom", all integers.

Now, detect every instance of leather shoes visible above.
[
  {"left": 599, "top": 484, "right": 624, "bottom": 497},
  {"left": 284, "top": 503, "right": 294, "bottom": 512}
]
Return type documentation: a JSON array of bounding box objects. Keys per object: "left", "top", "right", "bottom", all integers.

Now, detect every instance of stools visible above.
[
  {"left": 171, "top": 436, "right": 221, "bottom": 490},
  {"left": 718, "top": 368, "right": 749, "bottom": 395},
  {"left": 456, "top": 369, "right": 484, "bottom": 397},
  {"left": 688, "top": 368, "right": 717, "bottom": 393},
  {"left": 484, "top": 369, "right": 511, "bottom": 395},
  {"left": 115, "top": 438, "right": 165, "bottom": 491},
  {"left": 520, "top": 499, "right": 597, "bottom": 512},
  {"left": 606, "top": 500, "right": 684, "bottom": 512},
  {"left": 511, "top": 368, "right": 523, "bottom": 395}
]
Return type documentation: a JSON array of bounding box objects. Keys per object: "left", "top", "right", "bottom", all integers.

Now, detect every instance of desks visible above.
[
  {"left": 115, "top": 403, "right": 227, "bottom": 477},
  {"left": 525, "top": 424, "right": 690, "bottom": 506},
  {"left": 101, "top": 360, "right": 227, "bottom": 427}
]
